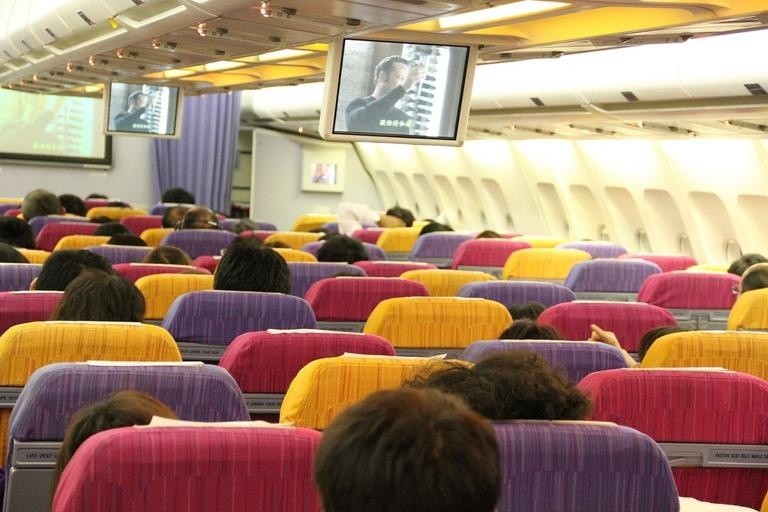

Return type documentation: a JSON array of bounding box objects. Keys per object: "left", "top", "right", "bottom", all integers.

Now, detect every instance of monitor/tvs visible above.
[
  {"left": 0, "top": 87, "right": 113, "bottom": 172},
  {"left": 319, "top": 28, "right": 479, "bottom": 148},
  {"left": 102, "top": 78, "right": 185, "bottom": 140}
]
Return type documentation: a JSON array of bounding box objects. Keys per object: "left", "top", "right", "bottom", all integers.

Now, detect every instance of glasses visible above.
[{"left": 729, "top": 284, "right": 741, "bottom": 295}]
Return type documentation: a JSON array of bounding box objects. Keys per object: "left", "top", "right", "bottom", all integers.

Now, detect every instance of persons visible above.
[
  {"left": 50, "top": 389, "right": 180, "bottom": 507},
  {"left": 728, "top": 253, "right": 768, "bottom": 299},
  {"left": 377, "top": 207, "right": 500, "bottom": 239},
  {"left": 0, "top": 188, "right": 366, "bottom": 323},
  {"left": 400, "top": 353, "right": 592, "bottom": 421},
  {"left": 313, "top": 387, "right": 499, "bottom": 512},
  {"left": 587, "top": 324, "right": 695, "bottom": 367},
  {"left": 114, "top": 91, "right": 155, "bottom": 132},
  {"left": 345, "top": 56, "right": 425, "bottom": 135},
  {"left": 508, "top": 300, "right": 547, "bottom": 322},
  {"left": 497, "top": 318, "right": 563, "bottom": 340}
]
